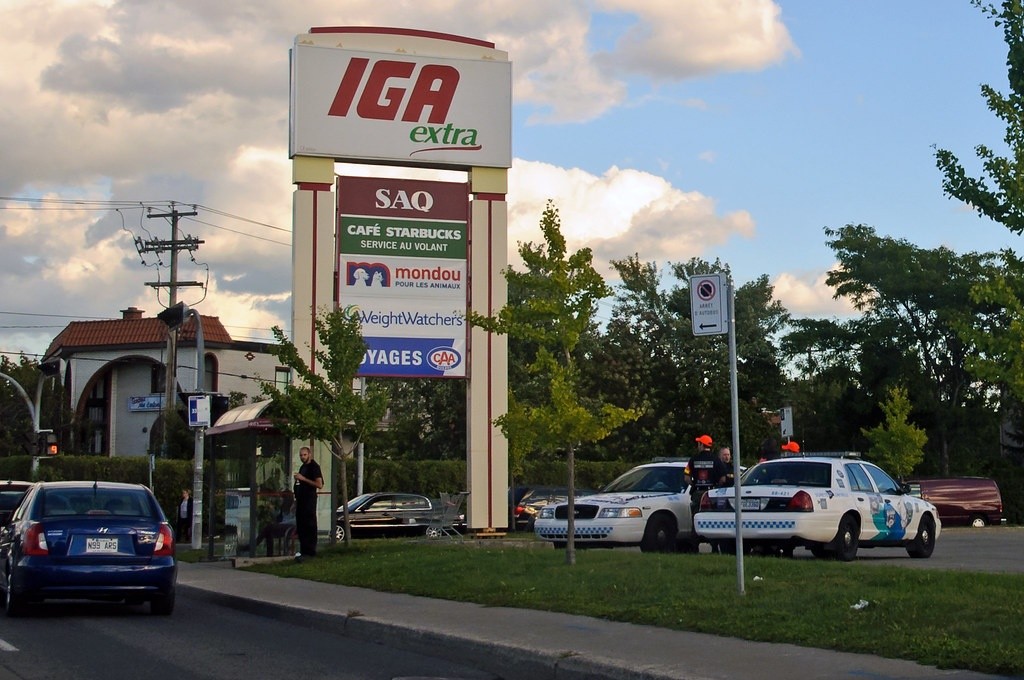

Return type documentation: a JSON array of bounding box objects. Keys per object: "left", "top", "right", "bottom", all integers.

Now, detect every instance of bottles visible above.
[{"left": 295, "top": 472, "right": 300, "bottom": 485}]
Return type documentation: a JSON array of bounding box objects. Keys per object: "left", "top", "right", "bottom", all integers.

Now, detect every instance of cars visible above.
[
  {"left": 0, "top": 480, "right": 179, "bottom": 617},
  {"left": 694, "top": 451, "right": 942, "bottom": 563},
  {"left": 534, "top": 457, "right": 771, "bottom": 554},
  {"left": 0, "top": 480, "right": 34, "bottom": 535},
  {"left": 330, "top": 491, "right": 476, "bottom": 542},
  {"left": 515, "top": 487, "right": 600, "bottom": 532}
]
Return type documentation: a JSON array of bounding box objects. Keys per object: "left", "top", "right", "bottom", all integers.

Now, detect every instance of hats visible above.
[
  {"left": 695, "top": 435, "right": 712, "bottom": 446},
  {"left": 782, "top": 442, "right": 800, "bottom": 452}
]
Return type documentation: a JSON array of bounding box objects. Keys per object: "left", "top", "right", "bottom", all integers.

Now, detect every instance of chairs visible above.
[
  {"left": 279, "top": 526, "right": 296, "bottom": 556},
  {"left": 774, "top": 474, "right": 799, "bottom": 484},
  {"left": 105, "top": 499, "right": 124, "bottom": 511}
]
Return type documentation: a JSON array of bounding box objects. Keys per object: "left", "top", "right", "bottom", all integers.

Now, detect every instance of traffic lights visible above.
[{"left": 46, "top": 431, "right": 60, "bottom": 457}]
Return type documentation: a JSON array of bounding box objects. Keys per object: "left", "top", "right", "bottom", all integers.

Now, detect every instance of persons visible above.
[
  {"left": 174, "top": 489, "right": 194, "bottom": 544},
  {"left": 238, "top": 489, "right": 296, "bottom": 558},
  {"left": 294, "top": 447, "right": 324, "bottom": 557},
  {"left": 684, "top": 435, "right": 799, "bottom": 558}
]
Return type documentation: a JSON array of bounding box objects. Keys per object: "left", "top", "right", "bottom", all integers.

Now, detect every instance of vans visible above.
[{"left": 897, "top": 475, "right": 1003, "bottom": 528}]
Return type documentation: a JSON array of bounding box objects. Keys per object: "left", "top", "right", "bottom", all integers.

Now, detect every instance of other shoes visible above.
[
  {"left": 176, "top": 539, "right": 180, "bottom": 543},
  {"left": 295, "top": 551, "right": 301, "bottom": 556},
  {"left": 237, "top": 544, "right": 251, "bottom": 552},
  {"left": 185, "top": 538, "right": 189, "bottom": 543}
]
